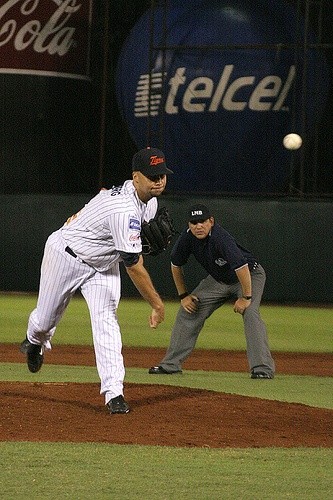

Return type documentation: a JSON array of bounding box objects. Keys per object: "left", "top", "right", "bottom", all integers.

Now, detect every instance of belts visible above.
[
  {"left": 252, "top": 262, "right": 257, "bottom": 272},
  {"left": 65, "top": 246, "right": 86, "bottom": 264}
]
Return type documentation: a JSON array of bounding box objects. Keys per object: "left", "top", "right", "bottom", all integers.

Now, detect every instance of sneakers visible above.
[
  {"left": 251, "top": 370, "right": 274, "bottom": 379},
  {"left": 20, "top": 334, "right": 44, "bottom": 373},
  {"left": 149, "top": 366, "right": 182, "bottom": 374},
  {"left": 107, "top": 395, "right": 129, "bottom": 414}
]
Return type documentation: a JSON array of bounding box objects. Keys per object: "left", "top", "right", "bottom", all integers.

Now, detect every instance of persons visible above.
[
  {"left": 25, "top": 147, "right": 174, "bottom": 414},
  {"left": 148, "top": 204, "right": 275, "bottom": 379}
]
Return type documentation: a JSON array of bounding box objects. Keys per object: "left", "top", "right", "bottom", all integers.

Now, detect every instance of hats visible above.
[
  {"left": 132, "top": 147, "right": 174, "bottom": 181},
  {"left": 188, "top": 204, "right": 210, "bottom": 221}
]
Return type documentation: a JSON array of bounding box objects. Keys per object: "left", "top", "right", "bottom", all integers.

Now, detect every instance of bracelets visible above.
[
  {"left": 239, "top": 294, "right": 253, "bottom": 300},
  {"left": 178, "top": 291, "right": 189, "bottom": 299}
]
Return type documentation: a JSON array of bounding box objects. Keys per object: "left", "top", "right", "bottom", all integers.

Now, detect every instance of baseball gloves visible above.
[{"left": 141, "top": 207, "right": 174, "bottom": 256}]
